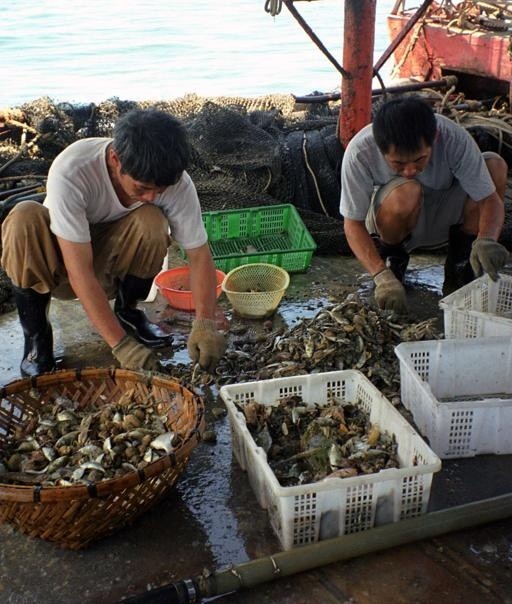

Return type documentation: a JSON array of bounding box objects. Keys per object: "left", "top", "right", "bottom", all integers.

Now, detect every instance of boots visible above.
[
  {"left": 12, "top": 284, "right": 55, "bottom": 377},
  {"left": 370, "top": 232, "right": 412, "bottom": 283},
  {"left": 442, "top": 223, "right": 484, "bottom": 298},
  {"left": 112, "top": 275, "right": 174, "bottom": 346}
]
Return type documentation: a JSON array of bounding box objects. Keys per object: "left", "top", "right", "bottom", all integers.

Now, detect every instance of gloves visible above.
[
  {"left": 112, "top": 336, "right": 162, "bottom": 371},
  {"left": 470, "top": 237, "right": 510, "bottom": 280},
  {"left": 188, "top": 320, "right": 228, "bottom": 371},
  {"left": 374, "top": 269, "right": 409, "bottom": 313}
]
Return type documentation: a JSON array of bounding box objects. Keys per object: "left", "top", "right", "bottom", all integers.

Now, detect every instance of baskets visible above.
[
  {"left": 438, "top": 272, "right": 512, "bottom": 338},
  {"left": 0, "top": 367, "right": 205, "bottom": 553},
  {"left": 201, "top": 203, "right": 317, "bottom": 274},
  {"left": 221, "top": 369, "right": 441, "bottom": 550},
  {"left": 221, "top": 262, "right": 290, "bottom": 320},
  {"left": 395, "top": 331, "right": 512, "bottom": 460},
  {"left": 155, "top": 266, "right": 225, "bottom": 311}
]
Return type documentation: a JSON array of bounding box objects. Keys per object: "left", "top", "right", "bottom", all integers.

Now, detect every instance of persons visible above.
[
  {"left": 340, "top": 85, "right": 511, "bottom": 324},
  {"left": 2, "top": 108, "right": 228, "bottom": 378}
]
